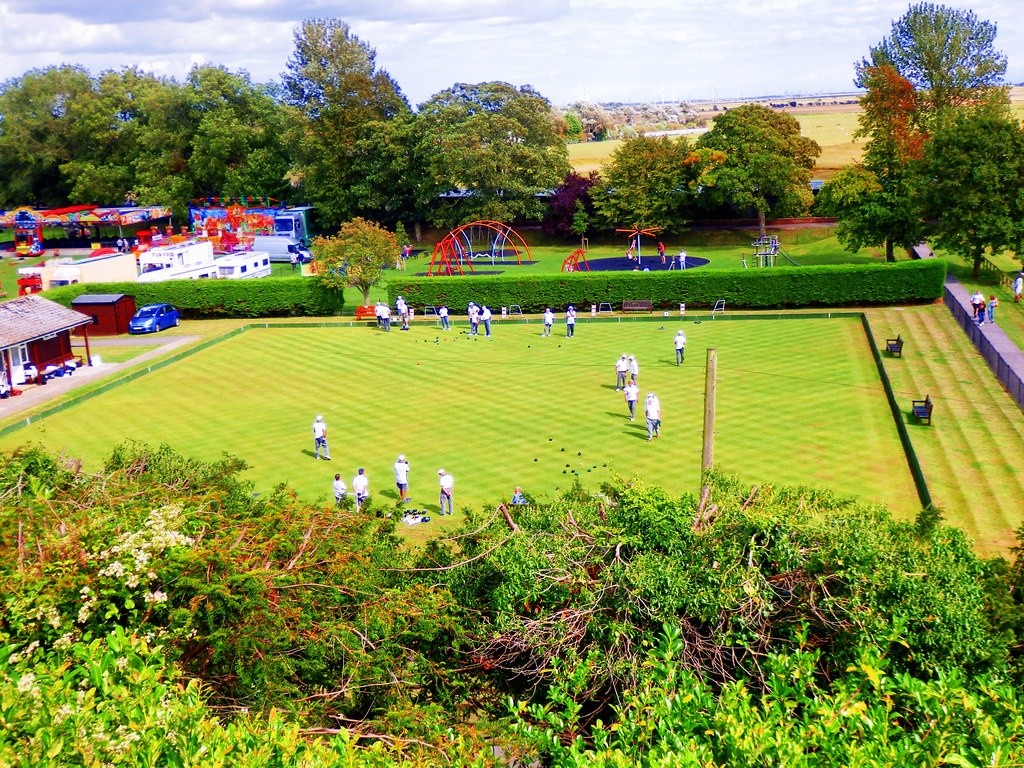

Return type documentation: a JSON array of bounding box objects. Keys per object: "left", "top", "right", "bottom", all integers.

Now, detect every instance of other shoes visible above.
[
  {"left": 647, "top": 438, "right": 652, "bottom": 442},
  {"left": 441, "top": 514, "right": 446, "bottom": 516},
  {"left": 449, "top": 513, "right": 452, "bottom": 515},
  {"left": 630, "top": 418, "right": 634, "bottom": 421},
  {"left": 622, "top": 387, "right": 626, "bottom": 390},
  {"left": 655, "top": 434, "right": 659, "bottom": 437},
  {"left": 615, "top": 388, "right": 620, "bottom": 391}
]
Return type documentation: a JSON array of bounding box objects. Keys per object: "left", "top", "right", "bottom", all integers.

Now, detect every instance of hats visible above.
[
  {"left": 358, "top": 468, "right": 365, "bottom": 473},
  {"left": 438, "top": 469, "right": 444, "bottom": 473},
  {"left": 316, "top": 416, "right": 323, "bottom": 421},
  {"left": 375, "top": 295, "right": 574, "bottom": 311},
  {"left": 399, "top": 455, "right": 406, "bottom": 461}
]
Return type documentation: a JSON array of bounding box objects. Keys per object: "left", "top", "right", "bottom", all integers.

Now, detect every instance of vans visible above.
[{"left": 254, "top": 235, "right": 313, "bottom": 263}]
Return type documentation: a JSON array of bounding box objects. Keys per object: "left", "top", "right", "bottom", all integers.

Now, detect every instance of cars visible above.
[{"left": 128, "top": 303, "right": 181, "bottom": 334}]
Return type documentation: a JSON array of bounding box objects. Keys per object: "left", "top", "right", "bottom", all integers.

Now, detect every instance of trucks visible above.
[
  {"left": 138, "top": 260, "right": 218, "bottom": 282},
  {"left": 272, "top": 206, "right": 320, "bottom": 238},
  {"left": 138, "top": 239, "right": 213, "bottom": 264},
  {"left": 41, "top": 252, "right": 138, "bottom": 289},
  {"left": 213, "top": 250, "right": 272, "bottom": 280}
]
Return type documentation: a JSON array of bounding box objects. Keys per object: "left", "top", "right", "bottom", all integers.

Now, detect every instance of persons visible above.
[
  {"left": 1014, "top": 272, "right": 1022, "bottom": 303},
  {"left": 480, "top": 306, "right": 492, "bottom": 336},
  {"left": 352, "top": 468, "right": 369, "bottom": 513},
  {"left": 644, "top": 391, "right": 661, "bottom": 431},
  {"left": 511, "top": 487, "right": 528, "bottom": 504},
  {"left": 313, "top": 416, "right": 332, "bottom": 460},
  {"left": 394, "top": 455, "right": 410, "bottom": 500},
  {"left": 439, "top": 305, "right": 450, "bottom": 330},
  {"left": 468, "top": 302, "right": 480, "bottom": 335},
  {"left": 615, "top": 354, "right": 629, "bottom": 392},
  {"left": 657, "top": 241, "right": 666, "bottom": 264},
  {"left": 679, "top": 250, "right": 686, "bottom": 270},
  {"left": 988, "top": 295, "right": 998, "bottom": 323},
  {"left": 565, "top": 306, "right": 577, "bottom": 338},
  {"left": 628, "top": 355, "right": 639, "bottom": 393},
  {"left": 471, "top": 310, "right": 480, "bottom": 335},
  {"left": 970, "top": 289, "right": 985, "bottom": 320},
  {"left": 674, "top": 331, "right": 686, "bottom": 366},
  {"left": 372, "top": 295, "right": 412, "bottom": 332},
  {"left": 624, "top": 380, "right": 639, "bottom": 421},
  {"left": 646, "top": 398, "right": 660, "bottom": 442},
  {"left": 975, "top": 301, "right": 986, "bottom": 327},
  {"left": 438, "top": 469, "right": 454, "bottom": 516},
  {"left": 541, "top": 308, "right": 554, "bottom": 337},
  {"left": 334, "top": 473, "right": 346, "bottom": 502}
]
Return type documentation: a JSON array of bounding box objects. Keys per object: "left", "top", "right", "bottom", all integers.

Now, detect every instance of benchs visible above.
[
  {"left": 912, "top": 394, "right": 933, "bottom": 426},
  {"left": 357, "top": 306, "right": 377, "bottom": 320},
  {"left": 622, "top": 299, "right": 653, "bottom": 314},
  {"left": 25, "top": 353, "right": 83, "bottom": 383},
  {"left": 886, "top": 334, "right": 904, "bottom": 357}
]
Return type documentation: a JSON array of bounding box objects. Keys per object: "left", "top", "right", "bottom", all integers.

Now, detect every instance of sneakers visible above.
[
  {"left": 324, "top": 455, "right": 331, "bottom": 460},
  {"left": 315, "top": 456, "right": 320, "bottom": 459},
  {"left": 377, "top": 324, "right": 573, "bottom": 339},
  {"left": 974, "top": 315, "right": 994, "bottom": 326}
]
